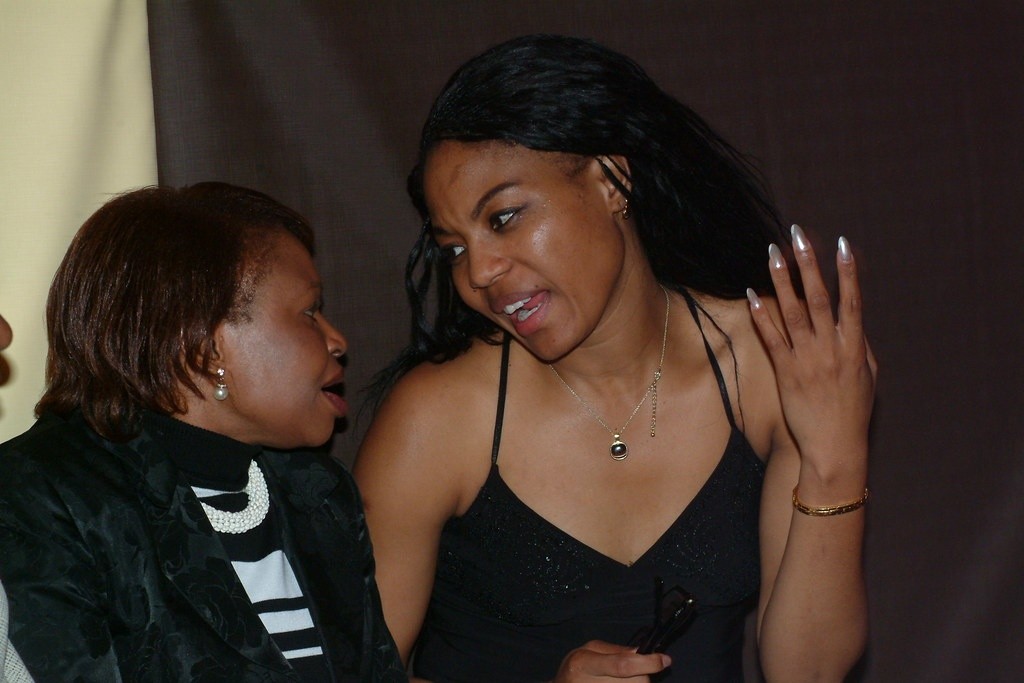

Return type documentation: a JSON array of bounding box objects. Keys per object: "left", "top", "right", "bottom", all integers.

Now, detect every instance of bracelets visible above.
[{"left": 788, "top": 478, "right": 871, "bottom": 518}]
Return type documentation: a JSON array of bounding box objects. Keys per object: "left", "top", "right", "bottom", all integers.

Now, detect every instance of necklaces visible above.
[
  {"left": 179, "top": 454, "right": 272, "bottom": 534},
  {"left": 541, "top": 281, "right": 672, "bottom": 461}
]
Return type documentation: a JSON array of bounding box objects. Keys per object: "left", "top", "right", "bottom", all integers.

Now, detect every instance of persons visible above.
[
  {"left": 345, "top": 27, "right": 885, "bottom": 683},
  {"left": 0, "top": 172, "right": 418, "bottom": 683}
]
